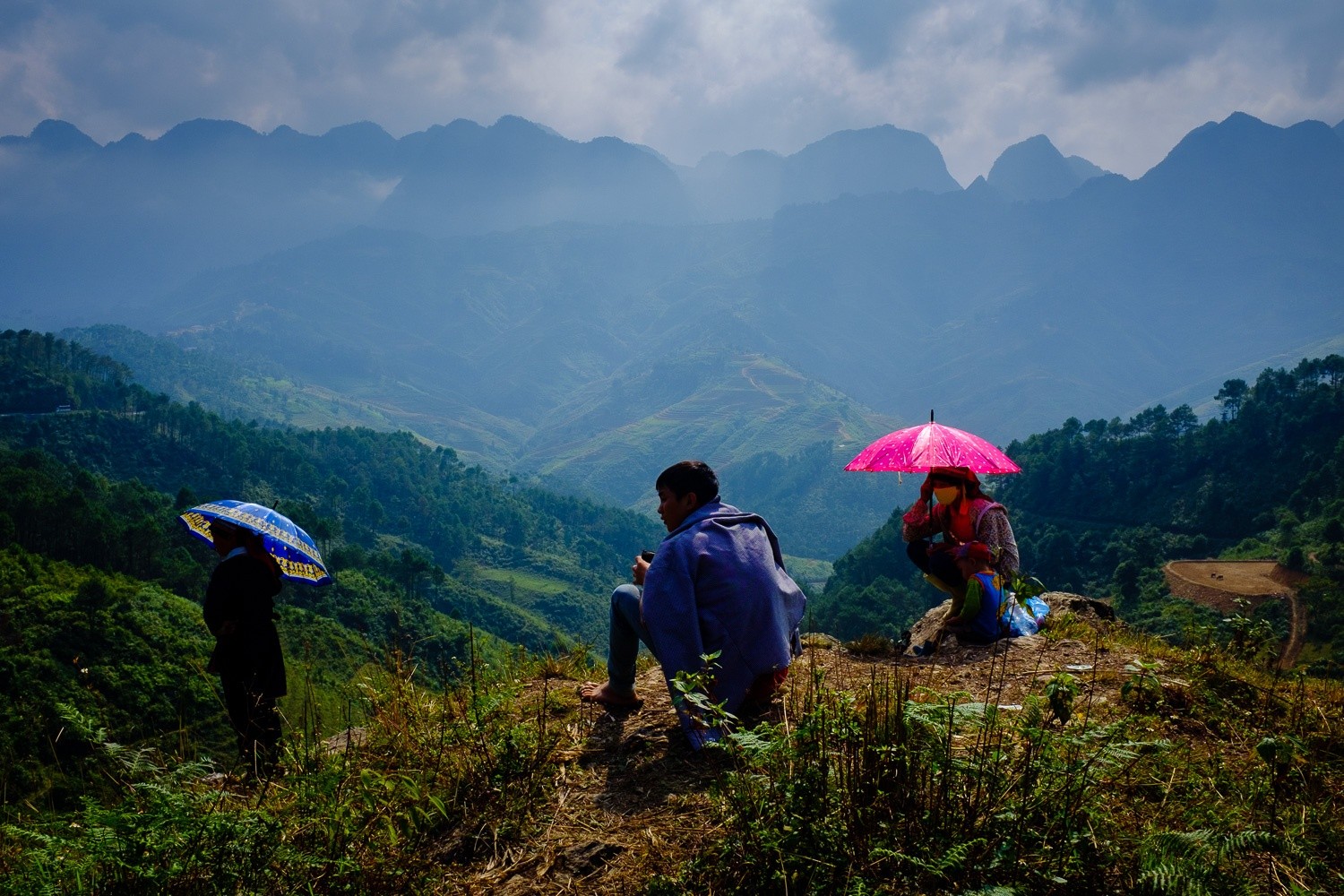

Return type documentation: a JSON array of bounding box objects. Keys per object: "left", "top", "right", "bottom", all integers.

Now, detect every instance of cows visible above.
[{"left": 1211, "top": 573, "right": 1224, "bottom": 581}]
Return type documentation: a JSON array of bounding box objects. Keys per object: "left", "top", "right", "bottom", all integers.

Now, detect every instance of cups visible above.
[{"left": 641, "top": 550, "right": 656, "bottom": 563}]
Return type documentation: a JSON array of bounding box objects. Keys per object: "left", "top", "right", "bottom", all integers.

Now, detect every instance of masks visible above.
[{"left": 933, "top": 485, "right": 959, "bottom": 505}]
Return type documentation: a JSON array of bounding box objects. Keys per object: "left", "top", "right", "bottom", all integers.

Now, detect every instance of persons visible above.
[
  {"left": 579, "top": 460, "right": 808, "bottom": 709},
  {"left": 202, "top": 518, "right": 287, "bottom": 786},
  {"left": 904, "top": 539, "right": 1006, "bottom": 659},
  {"left": 901, "top": 467, "right": 1020, "bottom": 622}
]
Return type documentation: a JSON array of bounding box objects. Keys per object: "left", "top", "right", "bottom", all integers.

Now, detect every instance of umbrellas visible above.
[
  {"left": 176, "top": 498, "right": 334, "bottom": 589},
  {"left": 841, "top": 408, "right": 1022, "bottom": 553}
]
